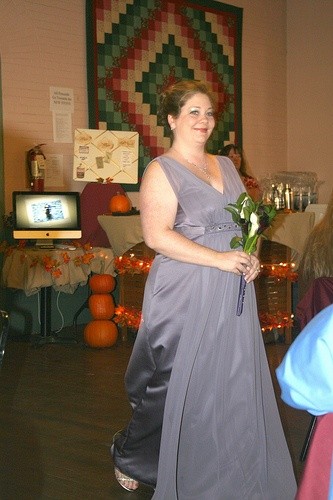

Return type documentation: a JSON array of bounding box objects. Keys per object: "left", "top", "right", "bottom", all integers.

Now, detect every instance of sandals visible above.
[{"left": 114, "top": 467, "right": 140, "bottom": 492}]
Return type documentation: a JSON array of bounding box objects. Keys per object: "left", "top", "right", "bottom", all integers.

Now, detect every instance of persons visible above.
[
  {"left": 275, "top": 304, "right": 333, "bottom": 500},
  {"left": 219, "top": 143, "right": 263, "bottom": 201},
  {"left": 110, "top": 78, "right": 298, "bottom": 500},
  {"left": 274, "top": 198, "right": 333, "bottom": 331}
]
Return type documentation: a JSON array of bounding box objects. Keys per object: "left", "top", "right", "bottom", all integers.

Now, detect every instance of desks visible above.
[
  {"left": 97, "top": 213, "right": 316, "bottom": 342},
  {"left": 0, "top": 242, "right": 116, "bottom": 350}
]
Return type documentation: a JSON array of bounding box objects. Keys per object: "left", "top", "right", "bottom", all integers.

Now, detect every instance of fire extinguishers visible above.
[{"left": 29, "top": 143, "right": 47, "bottom": 193}]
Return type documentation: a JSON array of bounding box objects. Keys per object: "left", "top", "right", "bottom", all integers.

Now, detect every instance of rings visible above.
[{"left": 256, "top": 268, "right": 260, "bottom": 272}]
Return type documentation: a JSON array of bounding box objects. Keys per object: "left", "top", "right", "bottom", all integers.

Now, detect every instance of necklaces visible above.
[{"left": 171, "top": 146, "right": 210, "bottom": 179}]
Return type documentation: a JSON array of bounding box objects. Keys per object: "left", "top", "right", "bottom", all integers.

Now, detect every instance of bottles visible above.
[
  {"left": 293, "top": 191, "right": 317, "bottom": 212},
  {"left": 282, "top": 183, "right": 292, "bottom": 210},
  {"left": 272, "top": 186, "right": 281, "bottom": 210}
]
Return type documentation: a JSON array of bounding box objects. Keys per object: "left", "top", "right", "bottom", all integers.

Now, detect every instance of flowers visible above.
[{"left": 225, "top": 191, "right": 278, "bottom": 253}]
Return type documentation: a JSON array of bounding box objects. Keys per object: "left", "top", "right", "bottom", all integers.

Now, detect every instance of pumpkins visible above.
[
  {"left": 110, "top": 192, "right": 130, "bottom": 212},
  {"left": 89, "top": 274, "right": 116, "bottom": 293},
  {"left": 84, "top": 320, "right": 118, "bottom": 347},
  {"left": 89, "top": 293, "right": 115, "bottom": 320}
]
